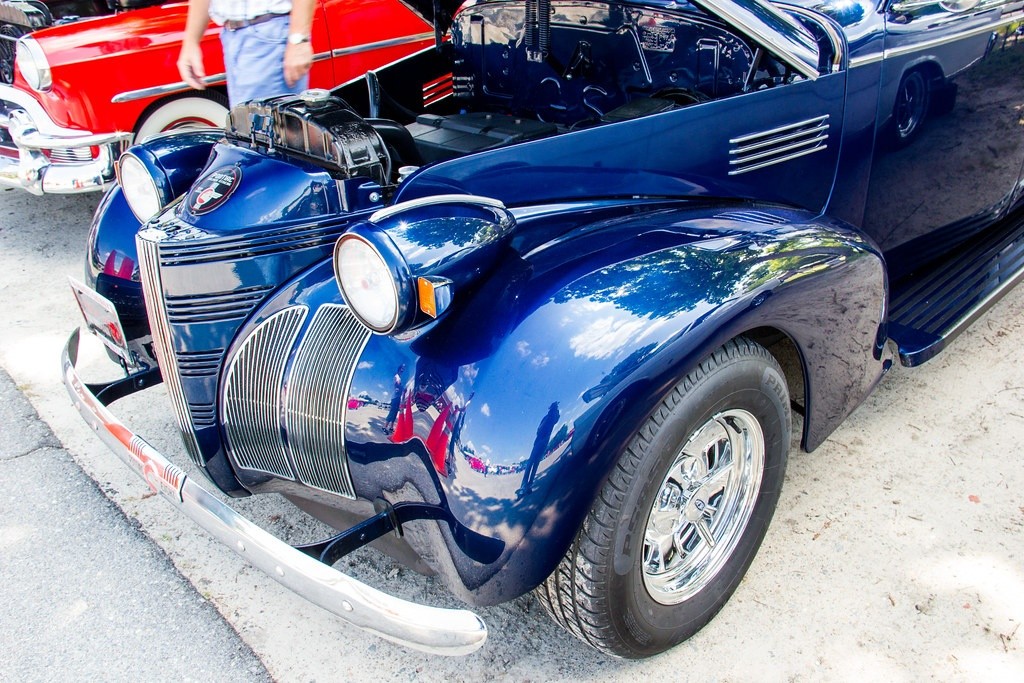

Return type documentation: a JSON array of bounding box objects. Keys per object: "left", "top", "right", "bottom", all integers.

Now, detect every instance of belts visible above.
[{"left": 224, "top": 12, "right": 290, "bottom": 31}]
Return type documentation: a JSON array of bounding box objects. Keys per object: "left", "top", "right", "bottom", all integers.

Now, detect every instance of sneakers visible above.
[
  {"left": 381, "top": 427, "right": 389, "bottom": 435},
  {"left": 389, "top": 428, "right": 394, "bottom": 433}
]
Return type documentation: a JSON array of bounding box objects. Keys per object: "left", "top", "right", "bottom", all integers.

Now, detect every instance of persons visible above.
[{"left": 177, "top": 0, "right": 317, "bottom": 112}]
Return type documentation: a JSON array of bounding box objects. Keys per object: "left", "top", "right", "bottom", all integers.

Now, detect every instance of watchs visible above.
[{"left": 287, "top": 34, "right": 310, "bottom": 45}]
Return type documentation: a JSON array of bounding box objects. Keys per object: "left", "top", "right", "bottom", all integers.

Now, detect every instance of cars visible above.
[
  {"left": 0, "top": 0, "right": 468, "bottom": 199},
  {"left": 48, "top": 0, "right": 1024, "bottom": 667}
]
